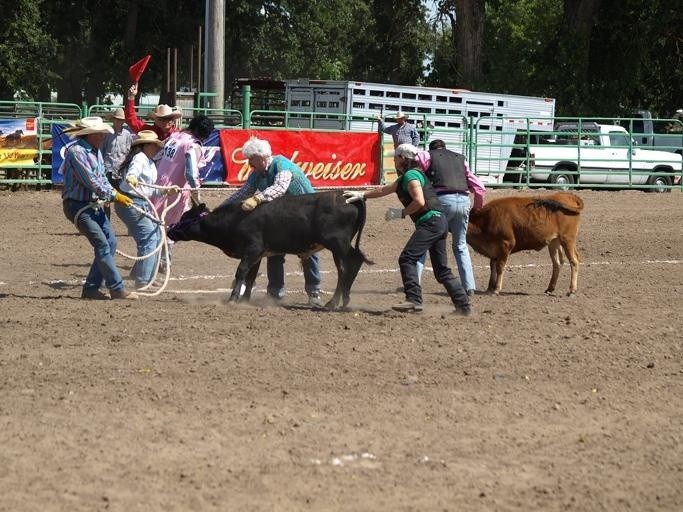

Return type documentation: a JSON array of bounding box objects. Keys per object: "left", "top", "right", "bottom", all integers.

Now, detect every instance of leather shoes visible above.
[
  {"left": 392, "top": 301, "right": 423, "bottom": 311},
  {"left": 450, "top": 310, "right": 472, "bottom": 317}
]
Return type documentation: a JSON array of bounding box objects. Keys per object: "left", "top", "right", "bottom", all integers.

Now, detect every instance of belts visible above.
[{"left": 437, "top": 190, "right": 469, "bottom": 197}]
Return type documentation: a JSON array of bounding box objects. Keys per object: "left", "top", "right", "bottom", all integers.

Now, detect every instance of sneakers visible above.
[
  {"left": 137, "top": 278, "right": 163, "bottom": 290},
  {"left": 308, "top": 295, "right": 322, "bottom": 308},
  {"left": 110, "top": 289, "right": 138, "bottom": 300},
  {"left": 82, "top": 289, "right": 109, "bottom": 300}
]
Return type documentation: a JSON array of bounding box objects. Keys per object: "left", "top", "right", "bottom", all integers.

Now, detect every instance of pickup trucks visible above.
[{"left": 517, "top": 121, "right": 683, "bottom": 193}]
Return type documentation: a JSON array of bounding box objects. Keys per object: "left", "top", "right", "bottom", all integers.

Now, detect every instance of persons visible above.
[
  {"left": 124, "top": 82, "right": 183, "bottom": 164},
  {"left": 104, "top": 108, "right": 132, "bottom": 222},
  {"left": 198, "top": 136, "right": 322, "bottom": 305},
  {"left": 148, "top": 115, "right": 215, "bottom": 274},
  {"left": 115, "top": 131, "right": 178, "bottom": 289},
  {"left": 417, "top": 140, "right": 486, "bottom": 297},
  {"left": 341, "top": 144, "right": 472, "bottom": 316},
  {"left": 62, "top": 116, "right": 139, "bottom": 299},
  {"left": 375, "top": 112, "right": 420, "bottom": 176}
]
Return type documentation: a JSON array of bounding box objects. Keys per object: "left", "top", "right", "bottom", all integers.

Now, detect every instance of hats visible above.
[
  {"left": 69, "top": 117, "right": 115, "bottom": 140},
  {"left": 132, "top": 130, "right": 161, "bottom": 145},
  {"left": 106, "top": 110, "right": 125, "bottom": 120},
  {"left": 149, "top": 105, "right": 182, "bottom": 119},
  {"left": 393, "top": 112, "right": 409, "bottom": 119}
]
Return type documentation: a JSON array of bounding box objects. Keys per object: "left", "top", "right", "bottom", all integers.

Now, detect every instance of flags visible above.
[{"left": 128, "top": 55, "right": 152, "bottom": 82}]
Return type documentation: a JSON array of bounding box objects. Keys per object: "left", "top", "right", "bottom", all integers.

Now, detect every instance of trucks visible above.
[{"left": 612, "top": 109, "right": 683, "bottom": 154}]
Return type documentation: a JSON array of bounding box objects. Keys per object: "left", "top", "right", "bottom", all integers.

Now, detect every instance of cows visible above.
[
  {"left": 448, "top": 192, "right": 584, "bottom": 297},
  {"left": 166, "top": 191, "right": 377, "bottom": 311}
]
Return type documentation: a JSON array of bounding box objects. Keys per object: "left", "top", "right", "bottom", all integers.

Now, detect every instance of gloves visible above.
[
  {"left": 241, "top": 195, "right": 261, "bottom": 211},
  {"left": 385, "top": 208, "right": 403, "bottom": 221},
  {"left": 342, "top": 191, "right": 365, "bottom": 204},
  {"left": 113, "top": 192, "right": 133, "bottom": 208},
  {"left": 126, "top": 176, "right": 137, "bottom": 186}
]
